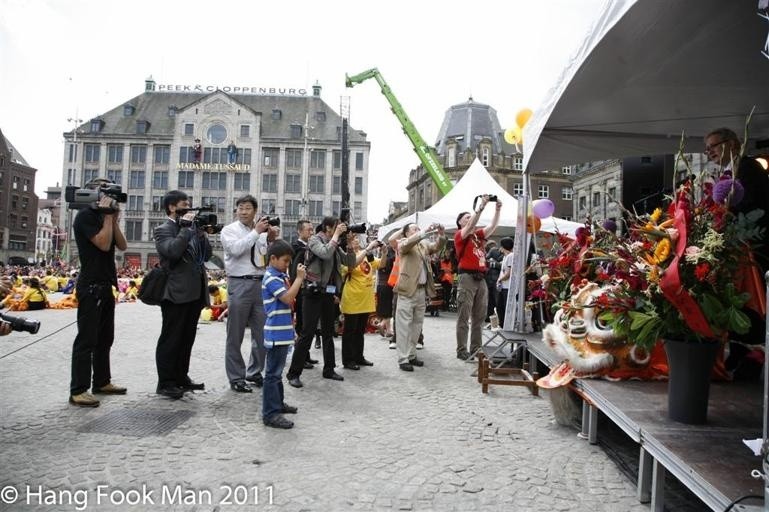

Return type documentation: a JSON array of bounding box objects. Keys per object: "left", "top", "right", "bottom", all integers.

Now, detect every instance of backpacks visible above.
[{"left": 138, "top": 263, "right": 168, "bottom": 304}]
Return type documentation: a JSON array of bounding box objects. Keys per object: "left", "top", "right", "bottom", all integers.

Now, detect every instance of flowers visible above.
[{"left": 578, "top": 99, "right": 769, "bottom": 349}]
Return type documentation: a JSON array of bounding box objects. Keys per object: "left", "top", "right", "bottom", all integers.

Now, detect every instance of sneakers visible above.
[
  {"left": 264, "top": 416, "right": 294, "bottom": 429},
  {"left": 416, "top": 344, "right": 422, "bottom": 348},
  {"left": 389, "top": 343, "right": 395, "bottom": 348},
  {"left": 282, "top": 403, "right": 298, "bottom": 413}
]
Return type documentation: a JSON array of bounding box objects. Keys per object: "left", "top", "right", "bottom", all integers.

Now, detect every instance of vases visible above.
[{"left": 659, "top": 337, "right": 723, "bottom": 429}]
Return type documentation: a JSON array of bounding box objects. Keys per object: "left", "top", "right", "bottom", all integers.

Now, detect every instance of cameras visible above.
[
  {"left": 258, "top": 216, "right": 279, "bottom": 232},
  {"left": 481, "top": 195, "right": 496, "bottom": 201},
  {"left": 1, "top": 312, "right": 40, "bottom": 334},
  {"left": 66, "top": 182, "right": 127, "bottom": 211},
  {"left": 335, "top": 221, "right": 366, "bottom": 240},
  {"left": 175, "top": 206, "right": 222, "bottom": 233}
]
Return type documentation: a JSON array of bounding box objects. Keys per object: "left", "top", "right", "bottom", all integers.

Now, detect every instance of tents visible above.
[{"left": 378, "top": 157, "right": 588, "bottom": 245}]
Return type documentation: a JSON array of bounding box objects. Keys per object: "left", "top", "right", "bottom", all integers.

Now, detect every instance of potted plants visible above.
[{"left": 535, "top": 214, "right": 610, "bottom": 317}]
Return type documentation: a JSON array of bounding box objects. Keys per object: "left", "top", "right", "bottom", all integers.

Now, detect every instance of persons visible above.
[
  {"left": 0, "top": 254, "right": 78, "bottom": 321},
  {"left": 0, "top": 318, "right": 12, "bottom": 336},
  {"left": 152, "top": 190, "right": 213, "bottom": 398},
  {"left": 117, "top": 260, "right": 144, "bottom": 303},
  {"left": 376, "top": 240, "right": 398, "bottom": 337},
  {"left": 209, "top": 283, "right": 232, "bottom": 322},
  {"left": 261, "top": 239, "right": 307, "bottom": 429},
  {"left": 339, "top": 232, "right": 388, "bottom": 371},
  {"left": 289, "top": 221, "right": 319, "bottom": 369},
  {"left": 388, "top": 239, "right": 424, "bottom": 349},
  {"left": 69, "top": 179, "right": 127, "bottom": 406},
  {"left": 485, "top": 241, "right": 501, "bottom": 321},
  {"left": 220, "top": 195, "right": 281, "bottom": 393},
  {"left": 706, "top": 128, "right": 769, "bottom": 383},
  {"left": 491, "top": 238, "right": 514, "bottom": 330},
  {"left": 454, "top": 194, "right": 502, "bottom": 360},
  {"left": 391, "top": 223, "right": 447, "bottom": 372},
  {"left": 286, "top": 217, "right": 356, "bottom": 388}
]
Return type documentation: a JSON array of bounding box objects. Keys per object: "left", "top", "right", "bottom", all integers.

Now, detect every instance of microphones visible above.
[{"left": 674, "top": 174, "right": 696, "bottom": 188}]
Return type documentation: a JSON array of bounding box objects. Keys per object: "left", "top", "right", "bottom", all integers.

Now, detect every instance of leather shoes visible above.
[
  {"left": 92, "top": 384, "right": 127, "bottom": 394},
  {"left": 345, "top": 363, "right": 359, "bottom": 369},
  {"left": 309, "top": 359, "right": 319, "bottom": 363},
  {"left": 357, "top": 359, "right": 373, "bottom": 366},
  {"left": 156, "top": 385, "right": 184, "bottom": 399},
  {"left": 70, "top": 392, "right": 101, "bottom": 407},
  {"left": 410, "top": 359, "right": 425, "bottom": 366},
  {"left": 400, "top": 363, "right": 414, "bottom": 371},
  {"left": 457, "top": 353, "right": 473, "bottom": 360},
  {"left": 232, "top": 381, "right": 252, "bottom": 392},
  {"left": 247, "top": 374, "right": 264, "bottom": 385},
  {"left": 471, "top": 350, "right": 484, "bottom": 359},
  {"left": 323, "top": 372, "right": 344, "bottom": 381},
  {"left": 286, "top": 374, "right": 303, "bottom": 388},
  {"left": 303, "top": 362, "right": 314, "bottom": 369},
  {"left": 176, "top": 378, "right": 205, "bottom": 389}
]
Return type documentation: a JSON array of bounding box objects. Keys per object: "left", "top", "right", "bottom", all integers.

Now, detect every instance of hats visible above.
[{"left": 456, "top": 212, "right": 470, "bottom": 229}]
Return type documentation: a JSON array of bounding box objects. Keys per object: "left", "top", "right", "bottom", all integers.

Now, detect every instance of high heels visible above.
[
  {"left": 376, "top": 323, "right": 384, "bottom": 337},
  {"left": 315, "top": 336, "right": 321, "bottom": 349}
]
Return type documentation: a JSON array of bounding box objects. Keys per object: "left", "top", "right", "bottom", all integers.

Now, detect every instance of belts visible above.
[
  {"left": 458, "top": 270, "right": 482, "bottom": 274},
  {"left": 228, "top": 275, "right": 264, "bottom": 279},
  {"left": 417, "top": 284, "right": 426, "bottom": 288}
]
